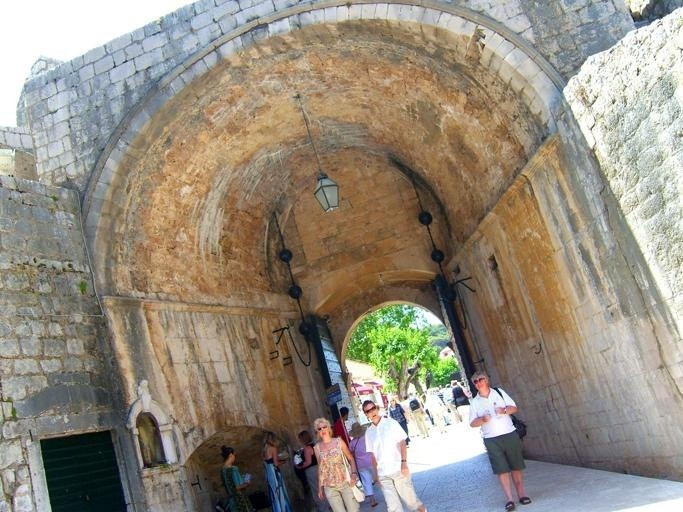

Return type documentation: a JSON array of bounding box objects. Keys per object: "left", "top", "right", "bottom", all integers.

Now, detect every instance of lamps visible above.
[{"left": 297, "top": 94, "right": 341, "bottom": 213}]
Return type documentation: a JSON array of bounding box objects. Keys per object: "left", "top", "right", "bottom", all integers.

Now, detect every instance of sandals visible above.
[
  {"left": 519, "top": 496, "right": 532, "bottom": 505},
  {"left": 504, "top": 500, "right": 515, "bottom": 511}
]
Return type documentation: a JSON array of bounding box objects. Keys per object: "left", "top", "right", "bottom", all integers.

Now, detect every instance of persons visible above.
[
  {"left": 221, "top": 445, "right": 256, "bottom": 512},
  {"left": 261, "top": 431, "right": 294, "bottom": 512},
  {"left": 469, "top": 370, "right": 531, "bottom": 511},
  {"left": 349, "top": 422, "right": 380, "bottom": 508},
  {"left": 362, "top": 400, "right": 427, "bottom": 512},
  {"left": 377, "top": 404, "right": 385, "bottom": 419},
  {"left": 294, "top": 431, "right": 332, "bottom": 512},
  {"left": 312, "top": 416, "right": 361, "bottom": 512},
  {"left": 388, "top": 376, "right": 474, "bottom": 448},
  {"left": 334, "top": 407, "right": 368, "bottom": 501}
]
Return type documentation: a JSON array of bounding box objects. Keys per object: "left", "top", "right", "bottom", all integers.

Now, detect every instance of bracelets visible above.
[
  {"left": 504, "top": 407, "right": 507, "bottom": 415},
  {"left": 401, "top": 460, "right": 408, "bottom": 463},
  {"left": 352, "top": 472, "right": 357, "bottom": 475}
]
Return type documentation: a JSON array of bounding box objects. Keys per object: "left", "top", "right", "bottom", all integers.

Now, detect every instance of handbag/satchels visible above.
[
  {"left": 293, "top": 453, "right": 303, "bottom": 466},
  {"left": 493, "top": 387, "right": 528, "bottom": 438},
  {"left": 346, "top": 465, "right": 366, "bottom": 502}
]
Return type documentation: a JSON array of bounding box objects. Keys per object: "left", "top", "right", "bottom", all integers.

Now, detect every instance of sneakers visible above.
[{"left": 371, "top": 502, "right": 378, "bottom": 508}]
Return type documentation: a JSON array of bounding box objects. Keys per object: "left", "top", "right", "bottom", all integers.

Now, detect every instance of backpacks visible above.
[{"left": 408, "top": 397, "right": 421, "bottom": 412}]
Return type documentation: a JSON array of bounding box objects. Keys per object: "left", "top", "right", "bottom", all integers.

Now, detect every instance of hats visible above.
[{"left": 349, "top": 422, "right": 367, "bottom": 439}]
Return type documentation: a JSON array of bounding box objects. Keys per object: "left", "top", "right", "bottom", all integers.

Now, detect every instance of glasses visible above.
[
  {"left": 365, "top": 406, "right": 377, "bottom": 414},
  {"left": 474, "top": 378, "right": 485, "bottom": 384},
  {"left": 317, "top": 426, "right": 328, "bottom": 430}
]
